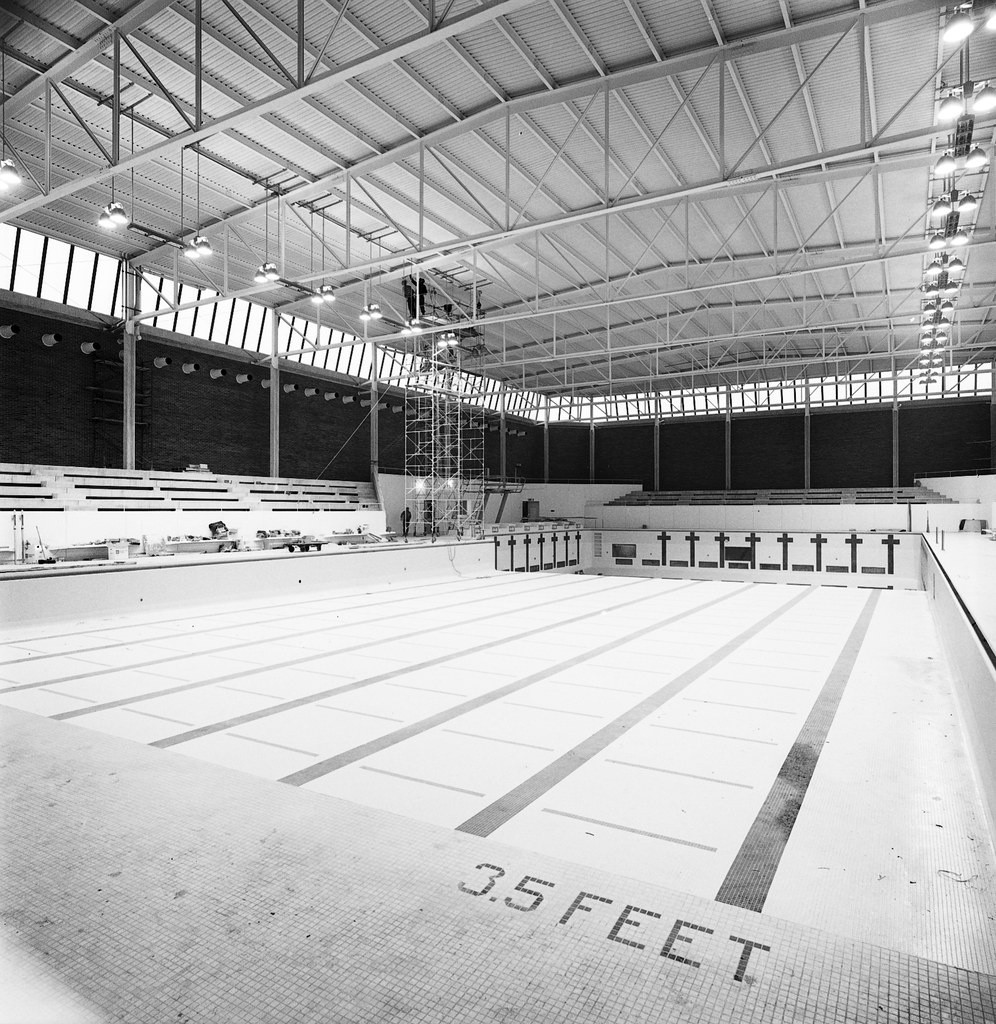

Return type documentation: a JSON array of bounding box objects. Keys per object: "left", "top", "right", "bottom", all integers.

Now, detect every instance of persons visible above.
[
  {"left": 427, "top": 503, "right": 437, "bottom": 533},
  {"left": 402, "top": 274, "right": 428, "bottom": 318},
  {"left": 400, "top": 507, "right": 412, "bottom": 537}
]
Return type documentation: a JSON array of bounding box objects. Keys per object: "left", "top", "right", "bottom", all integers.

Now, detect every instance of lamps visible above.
[
  {"left": 0, "top": 324, "right": 527, "bottom": 437},
  {"left": 921, "top": 0, "right": 996, "bottom": 385},
  {"left": 0, "top": 49, "right": 459, "bottom": 348}
]
